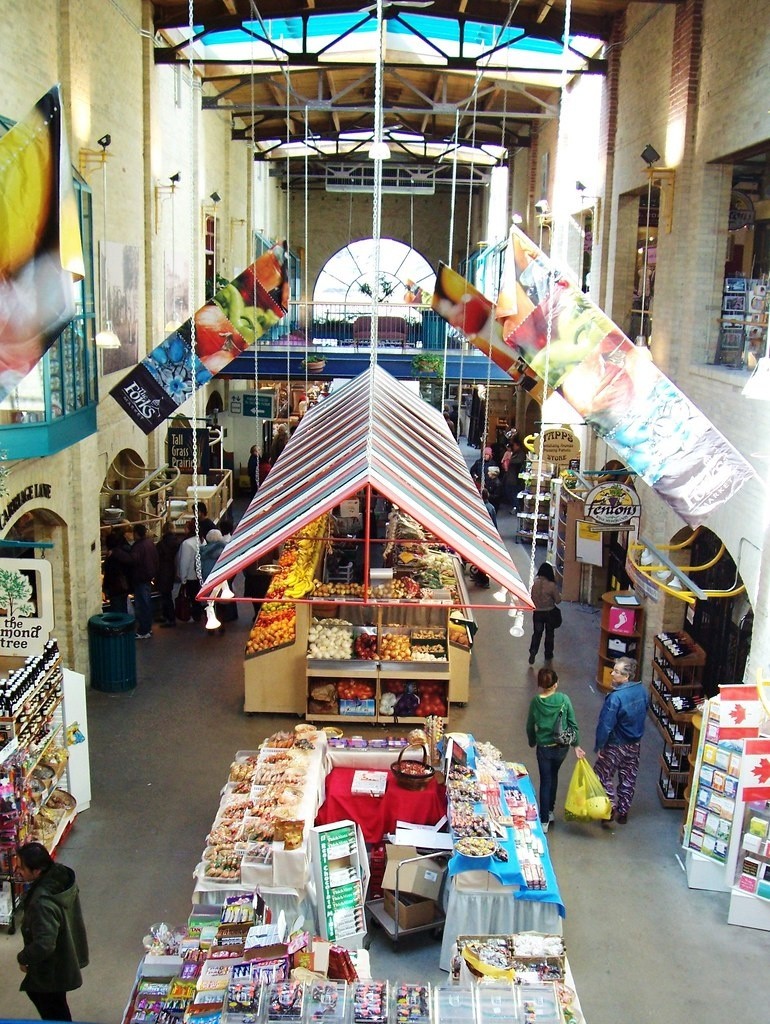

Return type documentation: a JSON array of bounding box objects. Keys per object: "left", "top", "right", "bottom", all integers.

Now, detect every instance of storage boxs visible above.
[
  {"left": 603, "top": 665, "right": 635, "bottom": 686},
  {"left": 606, "top": 607, "right": 638, "bottom": 634},
  {"left": 607, "top": 637, "right": 638, "bottom": 661},
  {"left": 305, "top": 595, "right": 457, "bottom": 728},
  {"left": 119, "top": 723, "right": 587, "bottom": 1024}
]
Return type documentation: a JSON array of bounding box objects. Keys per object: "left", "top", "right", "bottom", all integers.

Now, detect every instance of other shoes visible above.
[
  {"left": 159, "top": 620, "right": 176, "bottom": 628},
  {"left": 529, "top": 650, "right": 537, "bottom": 664},
  {"left": 618, "top": 812, "right": 628, "bottom": 824},
  {"left": 475, "top": 581, "right": 489, "bottom": 587},
  {"left": 545, "top": 652, "right": 554, "bottom": 659},
  {"left": 543, "top": 809, "right": 555, "bottom": 834},
  {"left": 602, "top": 805, "right": 618, "bottom": 825},
  {"left": 222, "top": 613, "right": 239, "bottom": 623},
  {"left": 135, "top": 630, "right": 153, "bottom": 638}
]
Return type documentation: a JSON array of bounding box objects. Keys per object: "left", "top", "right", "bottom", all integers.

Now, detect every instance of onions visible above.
[
  {"left": 380, "top": 633, "right": 412, "bottom": 661},
  {"left": 305, "top": 625, "right": 378, "bottom": 659},
  {"left": 194, "top": 304, "right": 248, "bottom": 376}
]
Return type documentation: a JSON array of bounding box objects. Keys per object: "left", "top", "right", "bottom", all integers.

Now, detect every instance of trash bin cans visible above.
[{"left": 89, "top": 612, "right": 136, "bottom": 692}]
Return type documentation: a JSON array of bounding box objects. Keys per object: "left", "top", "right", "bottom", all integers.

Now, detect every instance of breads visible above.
[{"left": 264, "top": 739, "right": 293, "bottom": 762}]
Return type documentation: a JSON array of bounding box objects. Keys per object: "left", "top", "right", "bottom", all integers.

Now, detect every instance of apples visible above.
[
  {"left": 245, "top": 615, "right": 295, "bottom": 655},
  {"left": 262, "top": 579, "right": 293, "bottom": 612}
]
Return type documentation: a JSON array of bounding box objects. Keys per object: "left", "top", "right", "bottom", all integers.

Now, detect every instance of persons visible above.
[
  {"left": 102, "top": 501, "right": 281, "bottom": 639},
  {"left": 594, "top": 658, "right": 650, "bottom": 825},
  {"left": 525, "top": 669, "right": 585, "bottom": 834},
  {"left": 527, "top": 562, "right": 561, "bottom": 664},
  {"left": 444, "top": 403, "right": 464, "bottom": 439},
  {"left": 468, "top": 432, "right": 526, "bottom": 531},
  {"left": 15, "top": 843, "right": 89, "bottom": 1022},
  {"left": 248, "top": 424, "right": 296, "bottom": 490}
]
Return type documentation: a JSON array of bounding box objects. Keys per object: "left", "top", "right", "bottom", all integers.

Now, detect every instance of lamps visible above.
[
  {"left": 155, "top": 172, "right": 186, "bottom": 335},
  {"left": 78, "top": 134, "right": 120, "bottom": 350},
  {"left": 533, "top": 199, "right": 555, "bottom": 250},
  {"left": 202, "top": 191, "right": 222, "bottom": 296},
  {"left": 575, "top": 181, "right": 602, "bottom": 294},
  {"left": 631, "top": 170, "right": 656, "bottom": 366},
  {"left": 741, "top": 300, "right": 769, "bottom": 401}
]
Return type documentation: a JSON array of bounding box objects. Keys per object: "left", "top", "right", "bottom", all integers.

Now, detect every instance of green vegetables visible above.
[{"left": 417, "top": 568, "right": 444, "bottom": 589}]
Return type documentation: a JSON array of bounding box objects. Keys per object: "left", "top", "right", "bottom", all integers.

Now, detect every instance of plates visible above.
[{"left": 454, "top": 840, "right": 497, "bottom": 858}]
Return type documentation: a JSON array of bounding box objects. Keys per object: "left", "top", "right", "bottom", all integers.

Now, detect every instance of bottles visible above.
[
  {"left": 646, "top": 632, "right": 705, "bottom": 800},
  {"left": 0, "top": 638, "right": 62, "bottom": 775}
]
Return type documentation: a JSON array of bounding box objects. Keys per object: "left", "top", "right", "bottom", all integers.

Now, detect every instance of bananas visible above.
[{"left": 279, "top": 512, "right": 327, "bottom": 598}]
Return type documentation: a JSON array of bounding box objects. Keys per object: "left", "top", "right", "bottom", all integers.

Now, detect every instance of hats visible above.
[
  {"left": 487, "top": 466, "right": 501, "bottom": 477},
  {"left": 485, "top": 448, "right": 493, "bottom": 457}
]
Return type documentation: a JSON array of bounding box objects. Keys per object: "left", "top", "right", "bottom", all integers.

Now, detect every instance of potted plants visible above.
[
  {"left": 413, "top": 352, "right": 449, "bottom": 389},
  {"left": 300, "top": 354, "right": 328, "bottom": 372}
]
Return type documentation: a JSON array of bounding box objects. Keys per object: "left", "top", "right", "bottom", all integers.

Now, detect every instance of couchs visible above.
[{"left": 353, "top": 316, "right": 406, "bottom": 350}]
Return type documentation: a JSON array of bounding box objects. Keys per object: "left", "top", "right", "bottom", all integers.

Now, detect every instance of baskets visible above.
[{"left": 391, "top": 742, "right": 434, "bottom": 791}]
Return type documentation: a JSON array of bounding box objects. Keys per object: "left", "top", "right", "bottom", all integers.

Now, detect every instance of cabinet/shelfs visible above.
[
  {"left": 553, "top": 494, "right": 585, "bottom": 600},
  {"left": 0, "top": 645, "right": 77, "bottom": 936},
  {"left": 730, "top": 739, "right": 770, "bottom": 903},
  {"left": 657, "top": 695, "right": 705, "bottom": 806},
  {"left": 515, "top": 464, "right": 555, "bottom": 544},
  {"left": 650, "top": 629, "right": 706, "bottom": 731},
  {"left": 681, "top": 697, "right": 770, "bottom": 868},
  {"left": 595, "top": 591, "right": 648, "bottom": 688}
]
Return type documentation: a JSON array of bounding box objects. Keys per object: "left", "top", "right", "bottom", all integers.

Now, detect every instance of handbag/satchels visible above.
[
  {"left": 175, "top": 585, "right": 191, "bottom": 621},
  {"left": 549, "top": 606, "right": 562, "bottom": 628},
  {"left": 563, "top": 756, "right": 612, "bottom": 823}
]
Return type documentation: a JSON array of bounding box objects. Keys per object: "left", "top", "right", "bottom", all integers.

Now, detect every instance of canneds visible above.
[{"left": 18, "top": 670, "right": 62, "bottom": 747}]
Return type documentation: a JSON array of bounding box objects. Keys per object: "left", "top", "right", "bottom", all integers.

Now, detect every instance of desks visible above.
[
  {"left": 439, "top": 753, "right": 566, "bottom": 975},
  {"left": 190, "top": 728, "right": 328, "bottom": 938},
  {"left": 317, "top": 769, "right": 449, "bottom": 839},
  {"left": 325, "top": 737, "right": 432, "bottom": 770}
]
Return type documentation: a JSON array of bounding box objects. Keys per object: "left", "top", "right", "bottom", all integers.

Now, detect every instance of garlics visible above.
[{"left": 412, "top": 652, "right": 448, "bottom": 661}]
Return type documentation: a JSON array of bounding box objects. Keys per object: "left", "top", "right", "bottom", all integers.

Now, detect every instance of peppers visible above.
[
  {"left": 529, "top": 294, "right": 616, "bottom": 388},
  {"left": 214, "top": 283, "right": 280, "bottom": 346}
]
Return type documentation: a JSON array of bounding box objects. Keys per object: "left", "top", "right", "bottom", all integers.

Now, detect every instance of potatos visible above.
[
  {"left": 312, "top": 578, "right": 409, "bottom": 598},
  {"left": 338, "top": 677, "right": 447, "bottom": 717}
]
